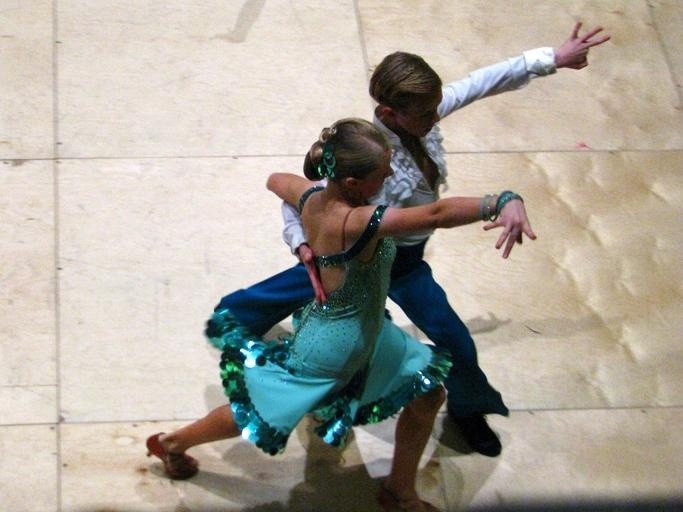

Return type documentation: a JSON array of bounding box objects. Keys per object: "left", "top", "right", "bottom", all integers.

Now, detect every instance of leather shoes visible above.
[
  {"left": 377, "top": 486, "right": 441, "bottom": 512},
  {"left": 447, "top": 397, "right": 502, "bottom": 458}
]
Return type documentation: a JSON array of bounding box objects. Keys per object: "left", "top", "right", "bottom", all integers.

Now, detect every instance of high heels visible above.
[{"left": 147, "top": 431, "right": 199, "bottom": 479}]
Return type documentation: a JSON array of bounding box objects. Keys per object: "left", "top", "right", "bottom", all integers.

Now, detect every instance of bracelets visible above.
[{"left": 479, "top": 189, "right": 524, "bottom": 223}]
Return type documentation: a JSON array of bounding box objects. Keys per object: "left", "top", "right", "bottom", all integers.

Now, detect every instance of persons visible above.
[
  {"left": 144, "top": 117, "right": 540, "bottom": 512},
  {"left": 278, "top": 19, "right": 617, "bottom": 457}
]
